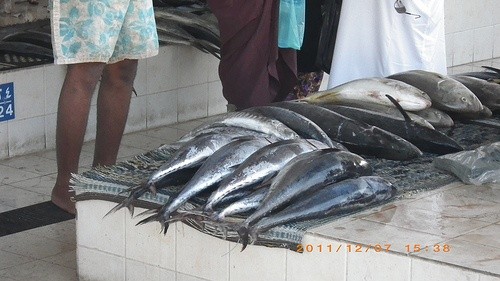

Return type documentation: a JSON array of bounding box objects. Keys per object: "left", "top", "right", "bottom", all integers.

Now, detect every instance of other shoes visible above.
[{"left": 52, "top": 191, "right": 76, "bottom": 215}]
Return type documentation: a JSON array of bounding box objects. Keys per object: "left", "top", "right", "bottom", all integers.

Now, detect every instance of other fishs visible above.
[
  {"left": 102, "top": 66, "right": 499, "bottom": 252},
  {"left": 1, "top": 0, "right": 222, "bottom": 96}
]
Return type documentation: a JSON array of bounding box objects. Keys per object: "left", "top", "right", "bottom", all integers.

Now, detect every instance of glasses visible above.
[{"left": 394, "top": 0, "right": 422, "bottom": 19}]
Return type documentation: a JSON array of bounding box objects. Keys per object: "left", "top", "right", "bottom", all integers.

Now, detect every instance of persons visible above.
[
  {"left": 47, "top": 0, "right": 159, "bottom": 214},
  {"left": 208, "top": 1, "right": 307, "bottom": 119},
  {"left": 316, "top": 0, "right": 448, "bottom": 91}
]
